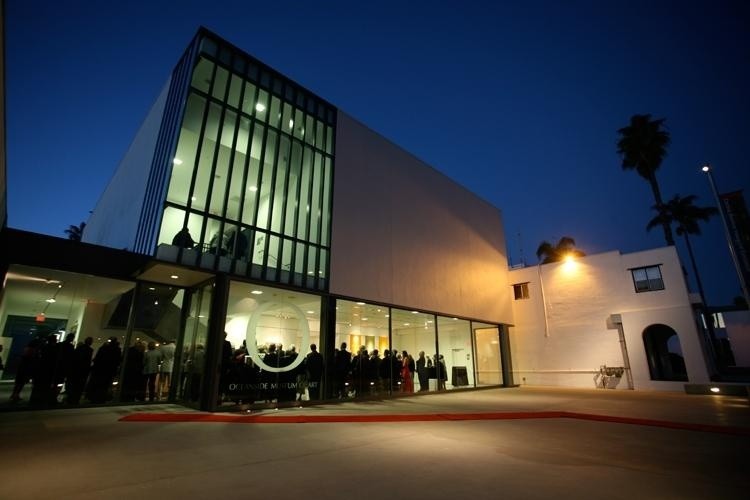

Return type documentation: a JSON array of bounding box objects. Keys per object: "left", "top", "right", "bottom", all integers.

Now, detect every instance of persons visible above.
[{"left": 171, "top": 227, "right": 228, "bottom": 256}]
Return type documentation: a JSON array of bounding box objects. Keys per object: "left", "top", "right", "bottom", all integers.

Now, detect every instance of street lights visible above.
[{"left": 700, "top": 160, "right": 750, "bottom": 307}]
